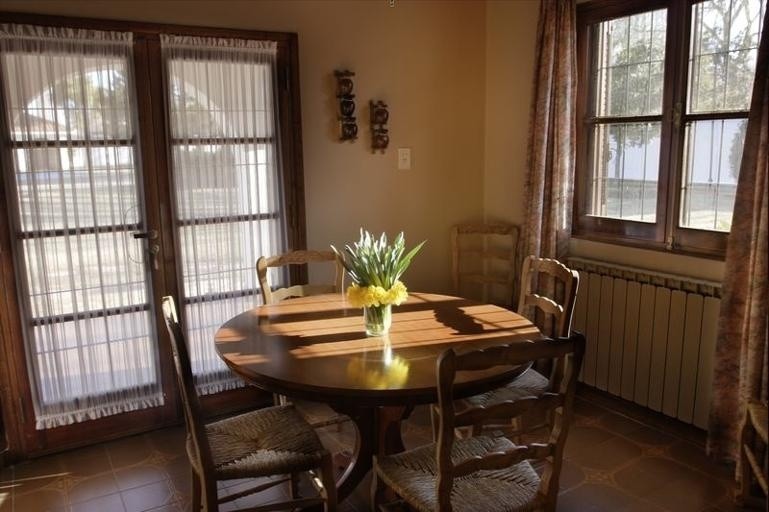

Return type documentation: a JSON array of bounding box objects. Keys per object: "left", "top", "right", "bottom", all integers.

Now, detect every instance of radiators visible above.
[{"left": 565, "top": 256, "right": 721, "bottom": 432}]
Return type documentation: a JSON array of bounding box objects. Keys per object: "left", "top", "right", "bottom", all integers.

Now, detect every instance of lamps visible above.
[
  {"left": 369, "top": 99, "right": 389, "bottom": 155},
  {"left": 333, "top": 70, "right": 359, "bottom": 140}
]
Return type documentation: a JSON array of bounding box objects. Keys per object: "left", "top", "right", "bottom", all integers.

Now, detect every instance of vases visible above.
[
  {"left": 363, "top": 305, "right": 392, "bottom": 336},
  {"left": 364, "top": 337, "right": 392, "bottom": 361}
]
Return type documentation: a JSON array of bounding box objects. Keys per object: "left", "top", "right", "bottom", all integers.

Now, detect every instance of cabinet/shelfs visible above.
[{"left": 449, "top": 224, "right": 519, "bottom": 312}]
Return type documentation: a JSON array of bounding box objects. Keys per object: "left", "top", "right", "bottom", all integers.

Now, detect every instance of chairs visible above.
[
  {"left": 367, "top": 329, "right": 585, "bottom": 512},
  {"left": 257, "top": 250, "right": 351, "bottom": 429},
  {"left": 162, "top": 294, "right": 337, "bottom": 512},
  {"left": 738, "top": 397, "right": 769, "bottom": 512},
  {"left": 430, "top": 255, "right": 580, "bottom": 447}
]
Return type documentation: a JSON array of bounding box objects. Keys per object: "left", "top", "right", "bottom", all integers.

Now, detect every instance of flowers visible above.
[
  {"left": 329, "top": 227, "right": 428, "bottom": 309},
  {"left": 346, "top": 355, "right": 410, "bottom": 389}
]
